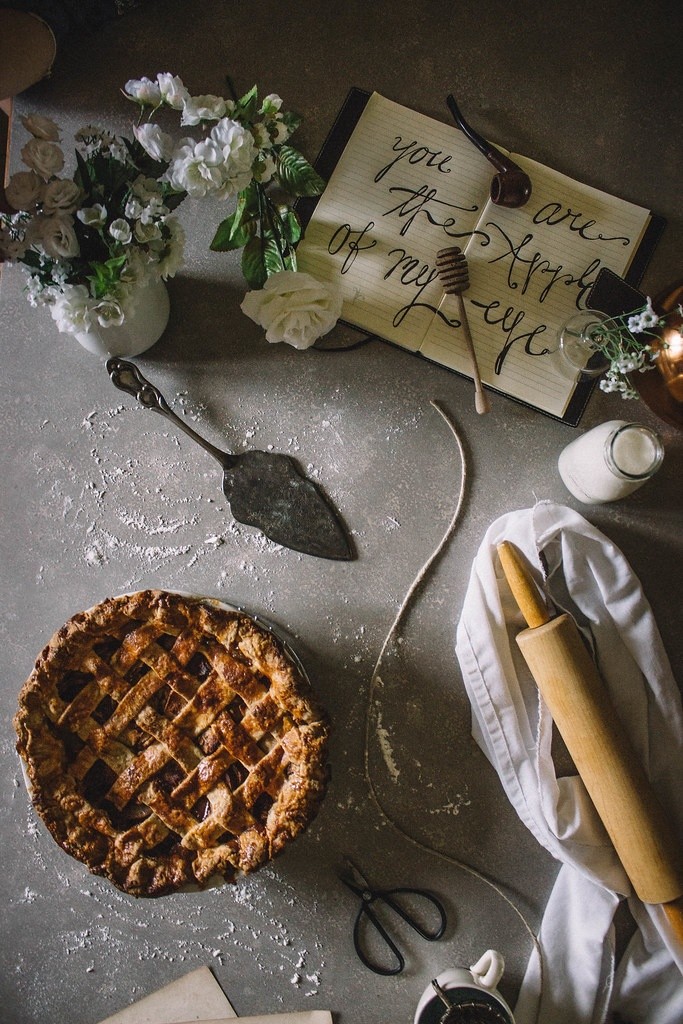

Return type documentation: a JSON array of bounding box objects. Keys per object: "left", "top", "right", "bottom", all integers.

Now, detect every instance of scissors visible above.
[{"left": 335, "top": 851, "right": 447, "bottom": 976}]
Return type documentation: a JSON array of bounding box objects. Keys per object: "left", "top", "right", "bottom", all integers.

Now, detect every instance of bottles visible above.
[{"left": 558, "top": 419, "right": 664, "bottom": 504}]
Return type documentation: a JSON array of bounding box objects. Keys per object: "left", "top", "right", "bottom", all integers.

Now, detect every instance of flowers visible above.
[
  {"left": 564, "top": 296, "right": 683, "bottom": 402},
  {"left": 0, "top": 72, "right": 260, "bottom": 334},
  {"left": 209, "top": 75, "right": 342, "bottom": 350}
]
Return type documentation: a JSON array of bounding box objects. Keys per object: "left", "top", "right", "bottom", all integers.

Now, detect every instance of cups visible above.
[{"left": 414, "top": 950, "right": 515, "bottom": 1024}]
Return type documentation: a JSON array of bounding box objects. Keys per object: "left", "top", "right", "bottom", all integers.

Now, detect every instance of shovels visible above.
[{"left": 105, "top": 357, "right": 355, "bottom": 562}]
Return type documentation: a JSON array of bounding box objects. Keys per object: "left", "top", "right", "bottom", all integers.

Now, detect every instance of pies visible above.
[{"left": 13, "top": 590, "right": 331, "bottom": 900}]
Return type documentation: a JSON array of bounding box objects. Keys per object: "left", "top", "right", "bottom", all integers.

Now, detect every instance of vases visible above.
[
  {"left": 45, "top": 253, "right": 169, "bottom": 359},
  {"left": 550, "top": 309, "right": 623, "bottom": 382}
]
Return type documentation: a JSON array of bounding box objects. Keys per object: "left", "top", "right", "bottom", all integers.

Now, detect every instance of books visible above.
[{"left": 277, "top": 86, "right": 665, "bottom": 427}]
